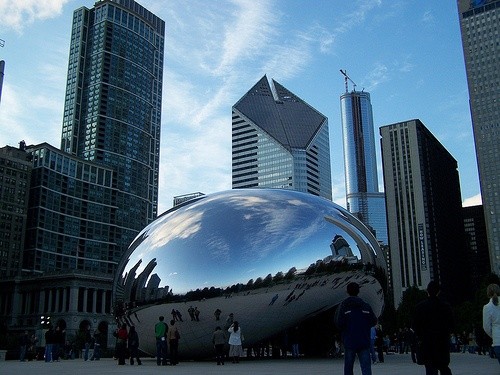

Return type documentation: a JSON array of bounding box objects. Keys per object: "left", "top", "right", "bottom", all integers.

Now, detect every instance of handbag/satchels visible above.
[
  {"left": 175, "top": 327, "right": 181, "bottom": 339},
  {"left": 241, "top": 334, "right": 244, "bottom": 341}
]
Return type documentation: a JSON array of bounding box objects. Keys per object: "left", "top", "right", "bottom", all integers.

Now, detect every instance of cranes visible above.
[{"left": 340, "top": 69, "right": 356, "bottom": 93}]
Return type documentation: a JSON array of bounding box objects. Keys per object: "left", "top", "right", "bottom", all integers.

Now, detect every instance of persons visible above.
[
  {"left": 128, "top": 326, "right": 141, "bottom": 365},
  {"left": 171, "top": 309, "right": 183, "bottom": 322},
  {"left": 44, "top": 325, "right": 66, "bottom": 362},
  {"left": 116, "top": 324, "right": 128, "bottom": 365},
  {"left": 84, "top": 323, "right": 91, "bottom": 361},
  {"left": 20, "top": 333, "right": 27, "bottom": 362},
  {"left": 228, "top": 321, "right": 242, "bottom": 363},
  {"left": 168, "top": 320, "right": 180, "bottom": 366},
  {"left": 370, "top": 326, "right": 384, "bottom": 364},
  {"left": 214, "top": 309, "right": 222, "bottom": 321},
  {"left": 89, "top": 329, "right": 102, "bottom": 361},
  {"left": 482, "top": 283, "right": 500, "bottom": 365},
  {"left": 247, "top": 326, "right": 300, "bottom": 358},
  {"left": 226, "top": 312, "right": 233, "bottom": 321},
  {"left": 188, "top": 305, "right": 200, "bottom": 322},
  {"left": 335, "top": 282, "right": 374, "bottom": 375},
  {"left": 211, "top": 326, "right": 227, "bottom": 365},
  {"left": 409, "top": 281, "right": 457, "bottom": 375},
  {"left": 154, "top": 316, "right": 170, "bottom": 366}
]
